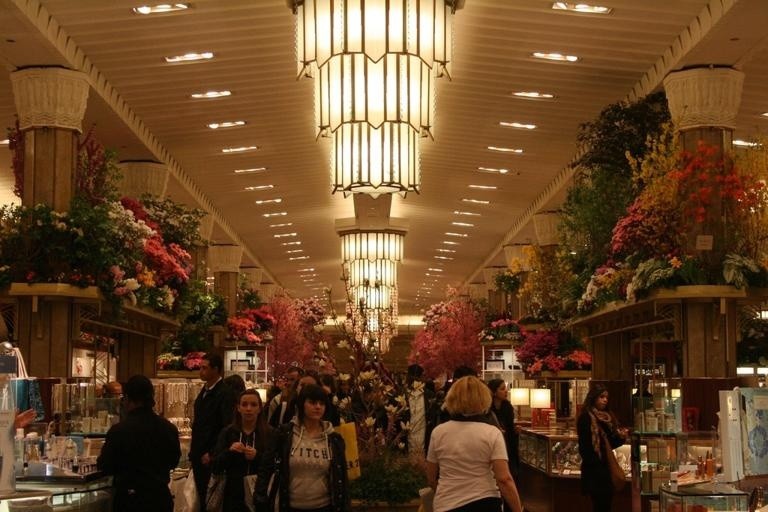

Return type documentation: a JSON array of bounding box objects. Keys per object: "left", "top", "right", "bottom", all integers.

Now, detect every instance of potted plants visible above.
[{"left": 347, "top": 461, "right": 434, "bottom": 512}]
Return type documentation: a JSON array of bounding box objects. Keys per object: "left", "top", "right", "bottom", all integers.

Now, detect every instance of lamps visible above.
[
  {"left": 334, "top": 227, "right": 408, "bottom": 357},
  {"left": 284, "top": 0, "right": 466, "bottom": 200}
]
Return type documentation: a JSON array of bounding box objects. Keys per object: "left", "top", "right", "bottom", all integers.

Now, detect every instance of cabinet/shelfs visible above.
[{"left": 516, "top": 426, "right": 750, "bottom": 512}]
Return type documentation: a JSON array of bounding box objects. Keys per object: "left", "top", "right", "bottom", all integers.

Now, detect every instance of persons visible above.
[
  {"left": 577, "top": 382, "right": 631, "bottom": 511},
  {"left": 94, "top": 375, "right": 183, "bottom": 511}
]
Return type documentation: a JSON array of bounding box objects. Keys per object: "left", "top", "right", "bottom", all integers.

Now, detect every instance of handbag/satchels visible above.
[
  {"left": 252, "top": 470, "right": 280, "bottom": 512},
  {"left": 333, "top": 422, "right": 361, "bottom": 481},
  {"left": 604, "top": 437, "right": 627, "bottom": 492},
  {"left": 203, "top": 471, "right": 227, "bottom": 512}
]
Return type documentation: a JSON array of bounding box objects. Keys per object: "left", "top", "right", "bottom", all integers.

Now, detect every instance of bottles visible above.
[{"left": 13, "top": 428, "right": 41, "bottom": 464}]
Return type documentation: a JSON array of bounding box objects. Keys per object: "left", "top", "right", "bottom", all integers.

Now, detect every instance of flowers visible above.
[
  {"left": 155, "top": 270, "right": 334, "bottom": 377},
  {"left": 289, "top": 255, "right": 460, "bottom": 470},
  {"left": 549, "top": 90, "right": 768, "bottom": 383},
  {"left": 403, "top": 243, "right": 550, "bottom": 383},
  {"left": 0, "top": 140, "right": 206, "bottom": 314}
]
